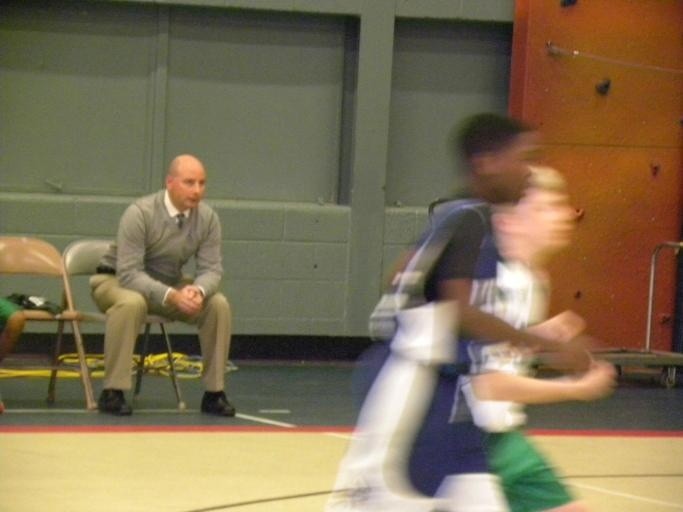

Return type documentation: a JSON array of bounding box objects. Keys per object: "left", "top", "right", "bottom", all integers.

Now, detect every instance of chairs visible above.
[
  {"left": 47, "top": 232, "right": 189, "bottom": 414},
  {"left": 0, "top": 231, "right": 98, "bottom": 410}
]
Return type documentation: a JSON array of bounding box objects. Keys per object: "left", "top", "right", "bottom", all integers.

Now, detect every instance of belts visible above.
[{"left": 96, "top": 266, "right": 116, "bottom": 275}]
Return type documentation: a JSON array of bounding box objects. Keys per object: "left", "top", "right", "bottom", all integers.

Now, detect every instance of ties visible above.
[{"left": 177, "top": 214, "right": 185, "bottom": 227}]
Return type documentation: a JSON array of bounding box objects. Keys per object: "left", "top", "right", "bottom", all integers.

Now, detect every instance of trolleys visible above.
[{"left": 526, "top": 239, "right": 682, "bottom": 388}]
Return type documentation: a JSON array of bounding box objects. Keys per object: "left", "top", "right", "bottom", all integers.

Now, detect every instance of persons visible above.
[
  {"left": 387, "top": 197, "right": 451, "bottom": 281},
  {"left": 469, "top": 166, "right": 618, "bottom": 512},
  {"left": 89, "top": 155, "right": 236, "bottom": 416},
  {"left": 0, "top": 298, "right": 25, "bottom": 416},
  {"left": 323, "top": 110, "right": 586, "bottom": 511}
]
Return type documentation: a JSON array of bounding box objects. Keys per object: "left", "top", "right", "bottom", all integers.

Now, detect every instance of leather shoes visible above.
[
  {"left": 202, "top": 391, "right": 235, "bottom": 416},
  {"left": 98, "top": 389, "right": 130, "bottom": 416}
]
